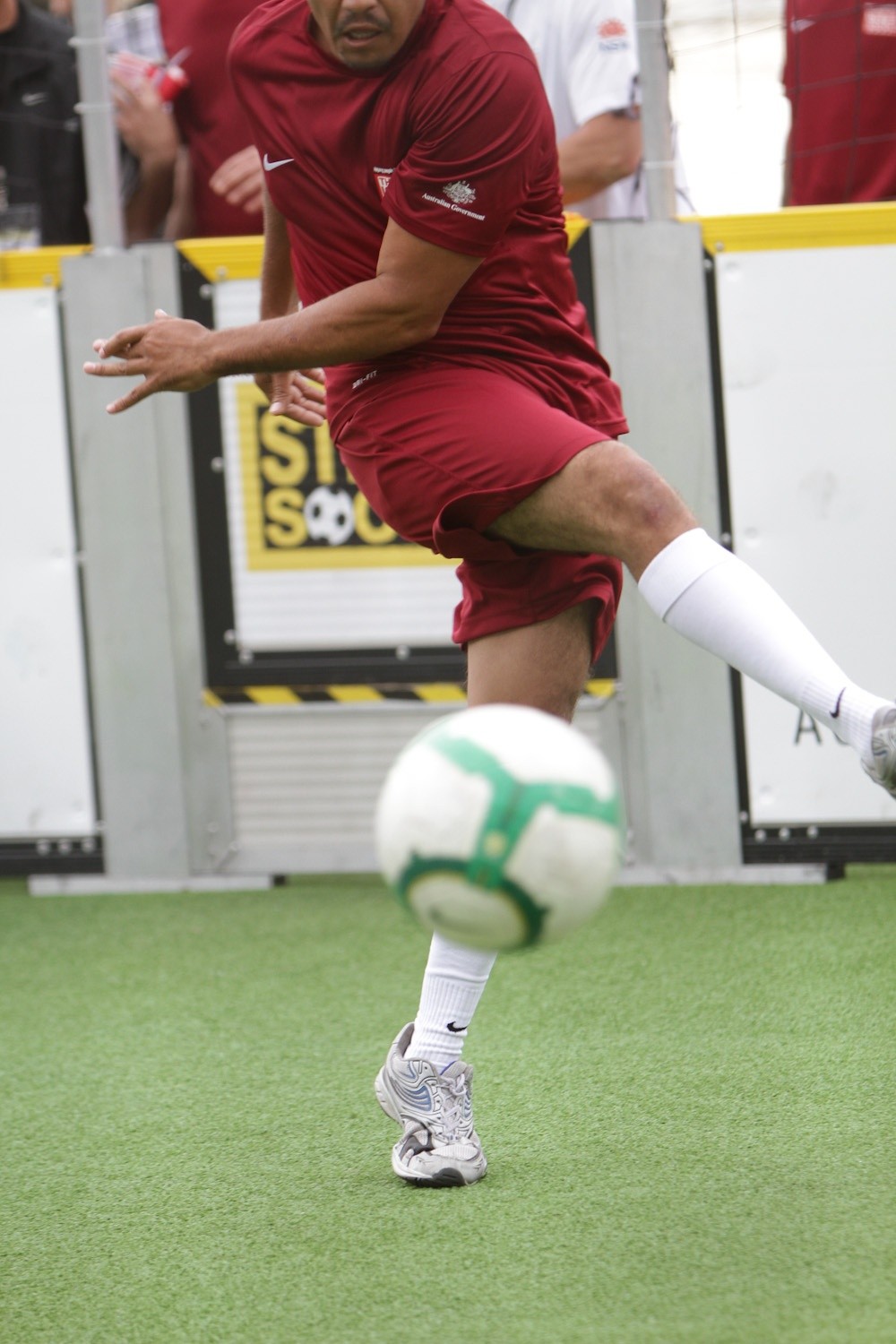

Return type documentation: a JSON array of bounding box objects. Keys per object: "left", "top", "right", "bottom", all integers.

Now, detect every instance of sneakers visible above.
[
  {"left": 375, "top": 1022, "right": 489, "bottom": 1186},
  {"left": 862, "top": 704, "right": 896, "bottom": 798}
]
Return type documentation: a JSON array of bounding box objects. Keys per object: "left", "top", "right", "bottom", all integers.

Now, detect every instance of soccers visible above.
[{"left": 368, "top": 702, "right": 622, "bottom": 953}]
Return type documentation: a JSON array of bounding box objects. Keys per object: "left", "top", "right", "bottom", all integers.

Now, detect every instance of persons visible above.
[
  {"left": 77, "top": 0, "right": 893, "bottom": 1192},
  {"left": 1, "top": 1, "right": 894, "bottom": 251}
]
